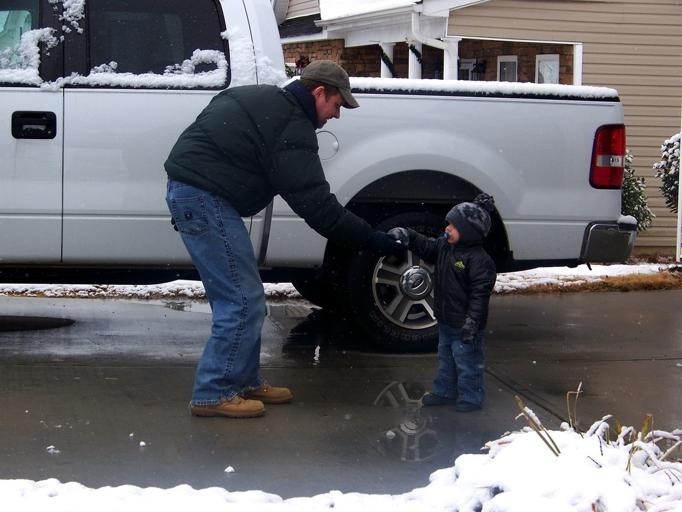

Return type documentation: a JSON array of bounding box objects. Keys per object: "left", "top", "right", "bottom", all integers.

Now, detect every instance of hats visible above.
[
  {"left": 301, "top": 60, "right": 358, "bottom": 108},
  {"left": 446, "top": 193, "right": 493, "bottom": 242}
]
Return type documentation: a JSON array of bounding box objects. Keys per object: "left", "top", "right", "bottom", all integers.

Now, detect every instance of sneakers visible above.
[
  {"left": 241, "top": 384, "right": 292, "bottom": 403},
  {"left": 191, "top": 396, "right": 263, "bottom": 417},
  {"left": 422, "top": 393, "right": 480, "bottom": 411}
]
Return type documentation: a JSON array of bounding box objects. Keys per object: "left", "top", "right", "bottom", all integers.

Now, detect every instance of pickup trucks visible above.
[{"left": 0, "top": 1, "right": 639, "bottom": 354}]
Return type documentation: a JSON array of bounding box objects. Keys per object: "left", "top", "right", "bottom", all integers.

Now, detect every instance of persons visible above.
[
  {"left": 162, "top": 59, "right": 411, "bottom": 418},
  {"left": 389, "top": 201, "right": 498, "bottom": 414}
]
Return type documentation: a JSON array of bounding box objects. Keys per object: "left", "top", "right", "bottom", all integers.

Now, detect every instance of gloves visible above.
[
  {"left": 462, "top": 320, "right": 479, "bottom": 344},
  {"left": 374, "top": 228, "right": 408, "bottom": 264}
]
